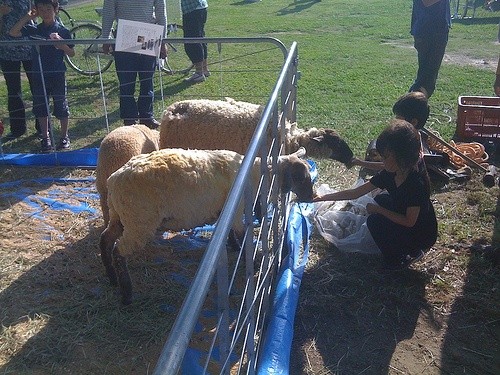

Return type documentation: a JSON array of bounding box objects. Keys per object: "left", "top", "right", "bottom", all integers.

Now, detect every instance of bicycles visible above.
[
  {"left": 63, "top": 8, "right": 196, "bottom": 76},
  {"left": 30, "top": 0, "right": 76, "bottom": 39}
]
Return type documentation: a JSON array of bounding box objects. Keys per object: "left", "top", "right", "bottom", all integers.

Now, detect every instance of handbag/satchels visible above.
[{"left": 315, "top": 179, "right": 382, "bottom": 255}]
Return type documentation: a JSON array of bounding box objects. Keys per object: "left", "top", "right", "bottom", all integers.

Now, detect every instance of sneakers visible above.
[
  {"left": 124, "top": 119, "right": 136, "bottom": 126},
  {"left": 41, "top": 138, "right": 54, "bottom": 152},
  {"left": 204, "top": 70, "right": 209, "bottom": 77},
  {"left": 184, "top": 74, "right": 206, "bottom": 82},
  {"left": 56, "top": 136, "right": 70, "bottom": 148},
  {"left": 387, "top": 248, "right": 424, "bottom": 273},
  {"left": 140, "top": 117, "right": 161, "bottom": 130}
]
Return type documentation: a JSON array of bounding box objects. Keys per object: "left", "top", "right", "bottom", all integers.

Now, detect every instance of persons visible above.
[
  {"left": 0, "top": 0, "right": 51, "bottom": 139},
  {"left": 180, "top": 0, "right": 211, "bottom": 83},
  {"left": 294, "top": 118, "right": 438, "bottom": 271},
  {"left": 11, "top": 0, "right": 75, "bottom": 149},
  {"left": 101, "top": 0, "right": 169, "bottom": 127},
  {"left": 408, "top": 0, "right": 451, "bottom": 99},
  {"left": 348, "top": 91, "right": 432, "bottom": 199}
]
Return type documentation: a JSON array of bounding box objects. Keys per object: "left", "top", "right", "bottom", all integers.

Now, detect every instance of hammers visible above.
[{"left": 422, "top": 127, "right": 496, "bottom": 188}]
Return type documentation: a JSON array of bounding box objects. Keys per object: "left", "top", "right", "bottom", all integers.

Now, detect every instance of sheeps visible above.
[
  {"left": 99, "top": 146, "right": 313, "bottom": 307},
  {"left": 95, "top": 98, "right": 354, "bottom": 225}
]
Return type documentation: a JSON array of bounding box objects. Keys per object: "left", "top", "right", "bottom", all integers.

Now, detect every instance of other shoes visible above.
[
  {"left": 6, "top": 127, "right": 26, "bottom": 138},
  {"left": 38, "top": 129, "right": 50, "bottom": 137}
]
